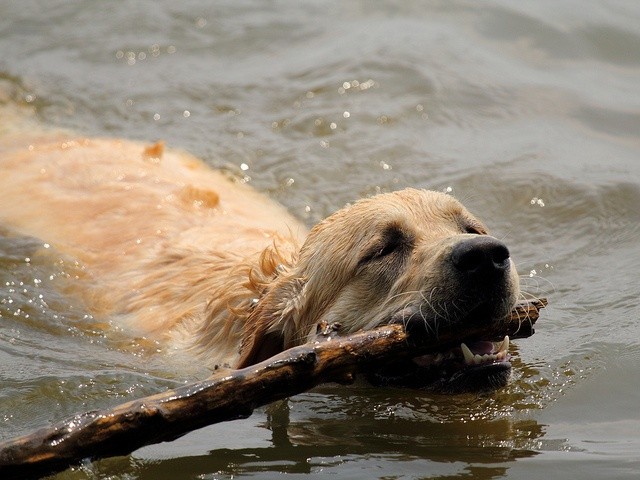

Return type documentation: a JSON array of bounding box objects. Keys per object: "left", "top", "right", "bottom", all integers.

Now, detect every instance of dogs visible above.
[{"left": 0, "top": 101, "right": 555, "bottom": 394}]
